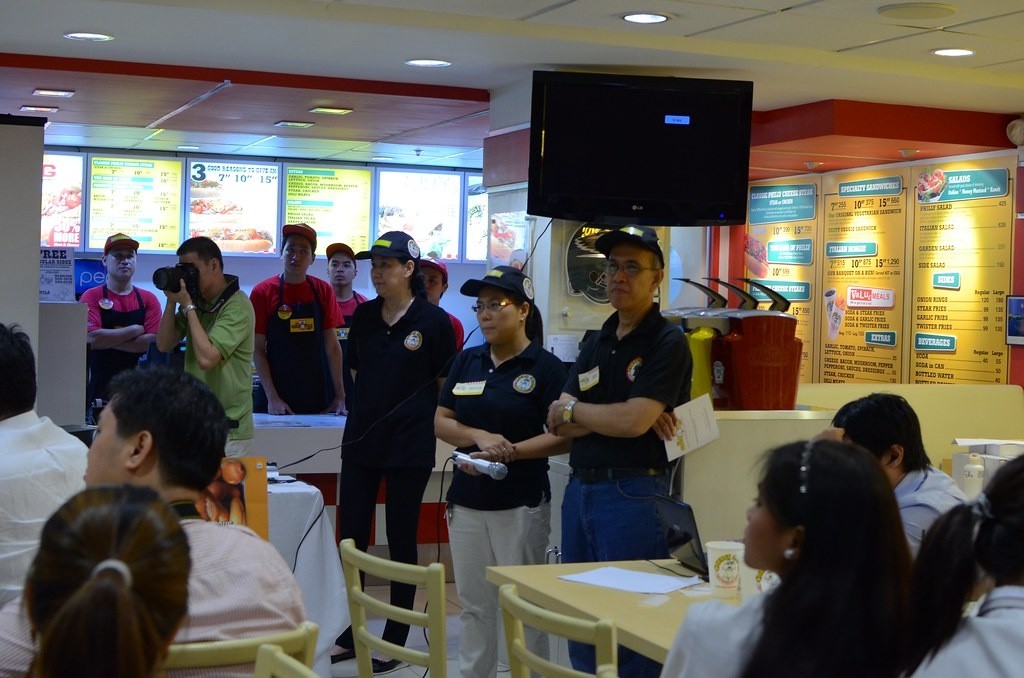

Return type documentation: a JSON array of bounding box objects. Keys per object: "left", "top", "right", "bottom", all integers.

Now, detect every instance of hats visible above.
[
  {"left": 281, "top": 222, "right": 317, "bottom": 246},
  {"left": 417, "top": 256, "right": 448, "bottom": 282},
  {"left": 325, "top": 241, "right": 356, "bottom": 265},
  {"left": 104, "top": 233, "right": 140, "bottom": 258},
  {"left": 354, "top": 230, "right": 422, "bottom": 267},
  {"left": 594, "top": 223, "right": 665, "bottom": 269},
  {"left": 459, "top": 264, "right": 535, "bottom": 308}
]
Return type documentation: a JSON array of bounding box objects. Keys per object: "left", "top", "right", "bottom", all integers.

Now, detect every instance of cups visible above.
[
  {"left": 706, "top": 539, "right": 747, "bottom": 599},
  {"left": 738, "top": 549, "right": 775, "bottom": 600}
]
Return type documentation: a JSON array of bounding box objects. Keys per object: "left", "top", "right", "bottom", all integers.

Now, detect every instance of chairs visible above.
[{"left": 143, "top": 536, "right": 621, "bottom": 678}]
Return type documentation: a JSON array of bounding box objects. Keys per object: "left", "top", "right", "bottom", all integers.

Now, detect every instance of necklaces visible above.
[
  {"left": 491, "top": 340, "right": 531, "bottom": 364},
  {"left": 381, "top": 294, "right": 415, "bottom": 323}
]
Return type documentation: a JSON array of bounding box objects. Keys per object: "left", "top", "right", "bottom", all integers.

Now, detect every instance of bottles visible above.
[{"left": 963, "top": 453, "right": 985, "bottom": 499}]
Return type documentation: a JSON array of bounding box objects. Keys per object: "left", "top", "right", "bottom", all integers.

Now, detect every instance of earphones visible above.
[{"left": 886, "top": 455, "right": 896, "bottom": 464}]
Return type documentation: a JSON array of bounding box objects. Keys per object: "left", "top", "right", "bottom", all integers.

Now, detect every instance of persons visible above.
[
  {"left": 652, "top": 432, "right": 924, "bottom": 678},
  {"left": 0, "top": 323, "right": 102, "bottom": 676},
  {"left": 75, "top": 233, "right": 164, "bottom": 426},
  {"left": 811, "top": 394, "right": 994, "bottom": 612},
  {"left": 319, "top": 231, "right": 460, "bottom": 675},
  {"left": 310, "top": 243, "right": 370, "bottom": 418},
  {"left": 0, "top": 482, "right": 199, "bottom": 678},
  {"left": 892, "top": 453, "right": 1024, "bottom": 678},
  {"left": 433, "top": 263, "right": 574, "bottom": 678},
  {"left": 548, "top": 227, "right": 697, "bottom": 677},
  {"left": 401, "top": 251, "right": 470, "bottom": 393},
  {"left": 245, "top": 223, "right": 353, "bottom": 419},
  {"left": 152, "top": 236, "right": 256, "bottom": 497},
  {"left": 1, "top": 363, "right": 319, "bottom": 678}
]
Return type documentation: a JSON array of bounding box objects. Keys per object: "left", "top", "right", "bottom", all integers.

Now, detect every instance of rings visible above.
[{"left": 501, "top": 448, "right": 507, "bottom": 454}]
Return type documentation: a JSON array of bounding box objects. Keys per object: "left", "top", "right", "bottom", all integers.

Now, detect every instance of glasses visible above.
[
  {"left": 600, "top": 259, "right": 661, "bottom": 278},
  {"left": 469, "top": 300, "right": 519, "bottom": 312}
]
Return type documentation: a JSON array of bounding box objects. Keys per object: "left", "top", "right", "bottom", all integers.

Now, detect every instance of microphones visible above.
[{"left": 450, "top": 451, "right": 507, "bottom": 480}]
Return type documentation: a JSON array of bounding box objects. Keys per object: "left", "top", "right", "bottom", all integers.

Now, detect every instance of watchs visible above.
[
  {"left": 562, "top": 394, "right": 580, "bottom": 423},
  {"left": 182, "top": 303, "right": 198, "bottom": 318}
]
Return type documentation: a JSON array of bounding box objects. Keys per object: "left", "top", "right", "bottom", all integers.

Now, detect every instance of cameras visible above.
[{"left": 152, "top": 262, "right": 199, "bottom": 293}]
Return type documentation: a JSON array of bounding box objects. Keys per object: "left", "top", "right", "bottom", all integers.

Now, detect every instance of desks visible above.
[
  {"left": 238, "top": 409, "right": 458, "bottom": 583},
  {"left": 264, "top": 464, "right": 347, "bottom": 678},
  {"left": 552, "top": 404, "right": 838, "bottom": 555},
  {"left": 497, "top": 559, "right": 717, "bottom": 678}
]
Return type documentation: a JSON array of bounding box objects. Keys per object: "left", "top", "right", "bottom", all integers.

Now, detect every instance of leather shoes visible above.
[
  {"left": 330, "top": 649, "right": 356, "bottom": 664},
  {"left": 371, "top": 656, "right": 401, "bottom": 674}
]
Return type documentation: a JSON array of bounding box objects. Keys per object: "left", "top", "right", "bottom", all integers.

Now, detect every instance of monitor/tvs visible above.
[{"left": 526, "top": 70, "right": 753, "bottom": 226}]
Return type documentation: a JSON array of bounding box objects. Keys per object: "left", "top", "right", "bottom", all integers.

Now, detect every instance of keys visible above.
[{"left": 440, "top": 502, "right": 455, "bottom": 525}]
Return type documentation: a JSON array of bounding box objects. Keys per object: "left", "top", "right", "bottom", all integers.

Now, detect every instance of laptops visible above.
[{"left": 651, "top": 492, "right": 710, "bottom": 583}]
